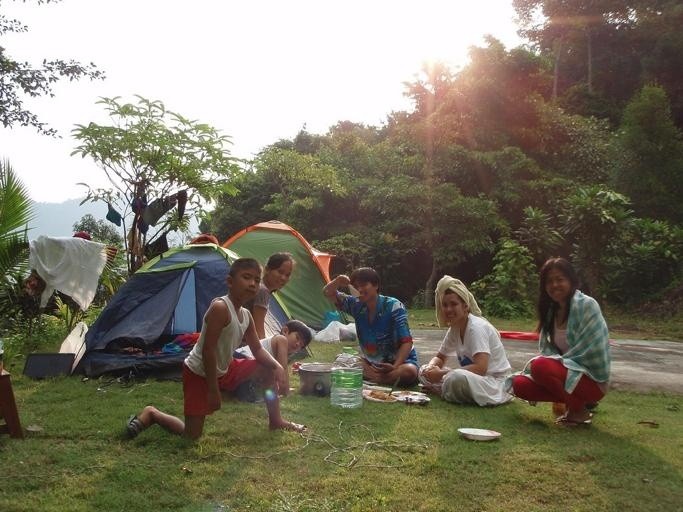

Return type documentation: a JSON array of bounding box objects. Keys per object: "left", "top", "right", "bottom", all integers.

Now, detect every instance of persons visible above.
[
  {"left": 320, "top": 266, "right": 422, "bottom": 389},
  {"left": 415, "top": 273, "right": 513, "bottom": 407},
  {"left": 123, "top": 257, "right": 307, "bottom": 442},
  {"left": 241, "top": 250, "right": 298, "bottom": 345},
  {"left": 229, "top": 318, "right": 313, "bottom": 404},
  {"left": 503, "top": 256, "right": 613, "bottom": 429}
]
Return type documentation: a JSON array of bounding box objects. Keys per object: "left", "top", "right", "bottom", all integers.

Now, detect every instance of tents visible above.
[
  {"left": 71, "top": 233, "right": 311, "bottom": 384},
  {"left": 220, "top": 220, "right": 349, "bottom": 333}
]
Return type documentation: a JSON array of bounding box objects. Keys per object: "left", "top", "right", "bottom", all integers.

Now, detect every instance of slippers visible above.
[
  {"left": 280, "top": 420, "right": 309, "bottom": 434},
  {"left": 125, "top": 411, "right": 147, "bottom": 437},
  {"left": 554, "top": 410, "right": 593, "bottom": 428}
]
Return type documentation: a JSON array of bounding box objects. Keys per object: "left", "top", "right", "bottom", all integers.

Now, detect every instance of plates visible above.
[
  {"left": 362, "top": 383, "right": 431, "bottom": 405},
  {"left": 456, "top": 427, "right": 503, "bottom": 442}
]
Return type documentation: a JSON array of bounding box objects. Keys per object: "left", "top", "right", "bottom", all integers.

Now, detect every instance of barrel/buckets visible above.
[
  {"left": 330, "top": 346, "right": 363, "bottom": 409},
  {"left": 298, "top": 361, "right": 340, "bottom": 395}
]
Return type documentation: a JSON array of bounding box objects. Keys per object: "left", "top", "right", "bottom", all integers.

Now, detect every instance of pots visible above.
[{"left": 292, "top": 360, "right": 343, "bottom": 397}]
